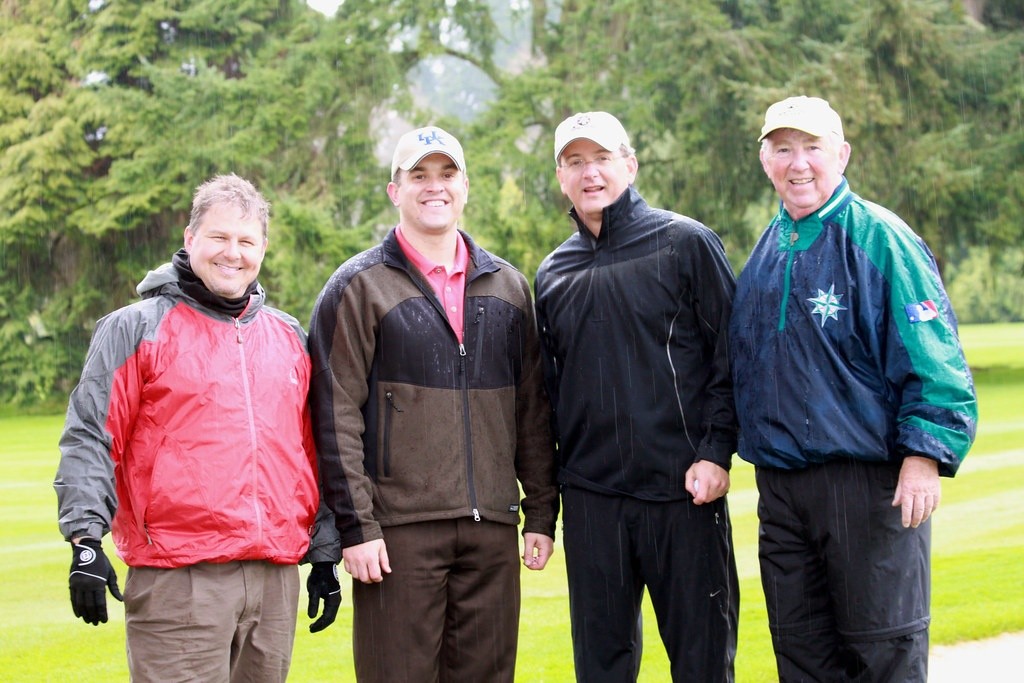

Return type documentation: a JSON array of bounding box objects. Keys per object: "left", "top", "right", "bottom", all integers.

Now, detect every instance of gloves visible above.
[
  {"left": 306, "top": 563, "right": 342, "bottom": 633},
  {"left": 68, "top": 538, "right": 125, "bottom": 625}
]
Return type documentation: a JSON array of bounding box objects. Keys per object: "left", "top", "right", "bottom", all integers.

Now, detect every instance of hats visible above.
[
  {"left": 756, "top": 95, "right": 846, "bottom": 143},
  {"left": 390, "top": 126, "right": 466, "bottom": 177},
  {"left": 553, "top": 111, "right": 630, "bottom": 164}
]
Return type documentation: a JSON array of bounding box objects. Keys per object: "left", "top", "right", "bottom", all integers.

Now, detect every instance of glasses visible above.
[{"left": 563, "top": 155, "right": 625, "bottom": 170}]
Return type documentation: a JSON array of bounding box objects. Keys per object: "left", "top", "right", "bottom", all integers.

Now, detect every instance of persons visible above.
[
  {"left": 534, "top": 111, "right": 741, "bottom": 683},
  {"left": 729, "top": 96, "right": 978, "bottom": 683},
  {"left": 52, "top": 172, "right": 341, "bottom": 683},
  {"left": 306, "top": 129, "right": 559, "bottom": 683}
]
